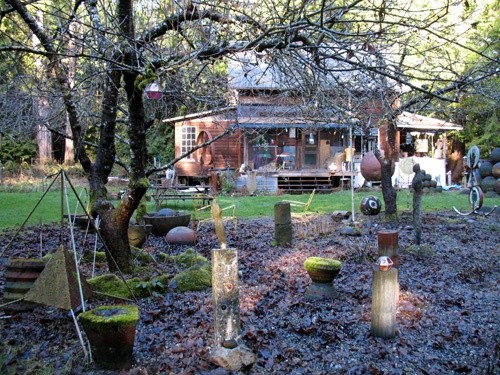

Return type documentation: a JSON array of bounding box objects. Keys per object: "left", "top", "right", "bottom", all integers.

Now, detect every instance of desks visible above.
[
  {"left": 277, "top": 154, "right": 296, "bottom": 170},
  {"left": 151, "top": 186, "right": 211, "bottom": 211}
]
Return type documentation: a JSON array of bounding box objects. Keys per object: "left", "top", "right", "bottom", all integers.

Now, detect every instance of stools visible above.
[{"left": 282, "top": 160, "right": 293, "bottom": 170}]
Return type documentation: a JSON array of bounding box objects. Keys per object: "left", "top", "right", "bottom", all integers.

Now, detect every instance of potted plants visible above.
[
  {"left": 77, "top": 304, "right": 140, "bottom": 371},
  {"left": 127, "top": 196, "right": 152, "bottom": 248},
  {"left": 304, "top": 257, "right": 343, "bottom": 299}
]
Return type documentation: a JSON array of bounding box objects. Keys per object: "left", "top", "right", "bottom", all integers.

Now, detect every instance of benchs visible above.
[{"left": 150, "top": 194, "right": 214, "bottom": 211}]
